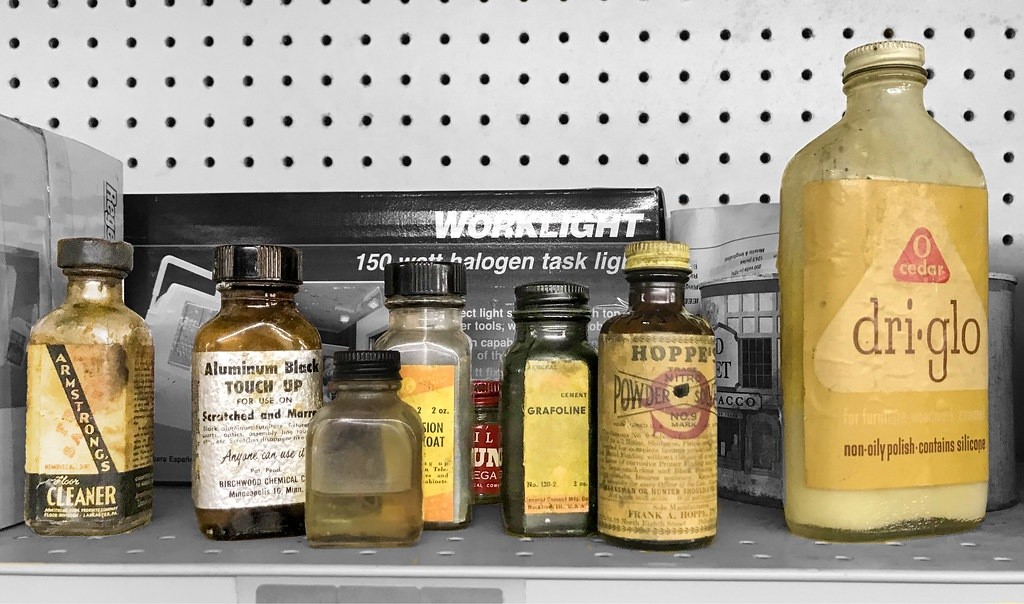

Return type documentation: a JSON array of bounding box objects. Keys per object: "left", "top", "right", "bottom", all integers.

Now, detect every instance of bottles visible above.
[
  {"left": 302, "top": 347, "right": 425, "bottom": 546},
  {"left": 468, "top": 376, "right": 504, "bottom": 509},
  {"left": 498, "top": 281, "right": 599, "bottom": 541},
  {"left": 372, "top": 257, "right": 475, "bottom": 529},
  {"left": 594, "top": 240, "right": 719, "bottom": 551},
  {"left": 22, "top": 235, "right": 155, "bottom": 536},
  {"left": 187, "top": 244, "right": 324, "bottom": 541},
  {"left": 772, "top": 36, "right": 989, "bottom": 546}
]
